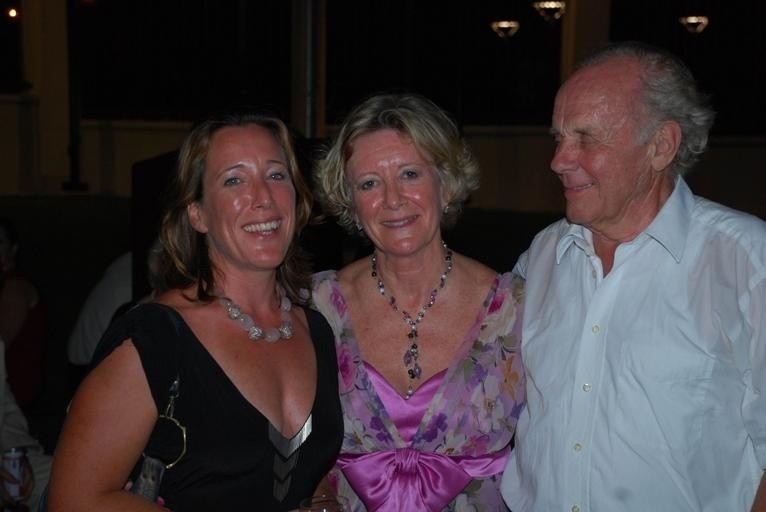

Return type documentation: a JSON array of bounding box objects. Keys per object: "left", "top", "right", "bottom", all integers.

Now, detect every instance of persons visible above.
[
  {"left": 44, "top": 115, "right": 344, "bottom": 512},
  {"left": 0, "top": 338, "right": 52, "bottom": 512},
  {"left": 301, "top": 90, "right": 527, "bottom": 512},
  {"left": 499, "top": 43, "right": 766, "bottom": 512},
  {"left": 0, "top": 217, "right": 49, "bottom": 411},
  {"left": 66, "top": 249, "right": 133, "bottom": 367}
]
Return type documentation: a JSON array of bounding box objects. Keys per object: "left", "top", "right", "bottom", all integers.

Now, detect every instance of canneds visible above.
[{"left": 3, "top": 446, "right": 26, "bottom": 501}]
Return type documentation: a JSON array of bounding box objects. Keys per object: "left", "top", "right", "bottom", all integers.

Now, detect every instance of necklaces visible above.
[
  {"left": 372, "top": 240, "right": 453, "bottom": 400},
  {"left": 215, "top": 288, "right": 295, "bottom": 343}
]
